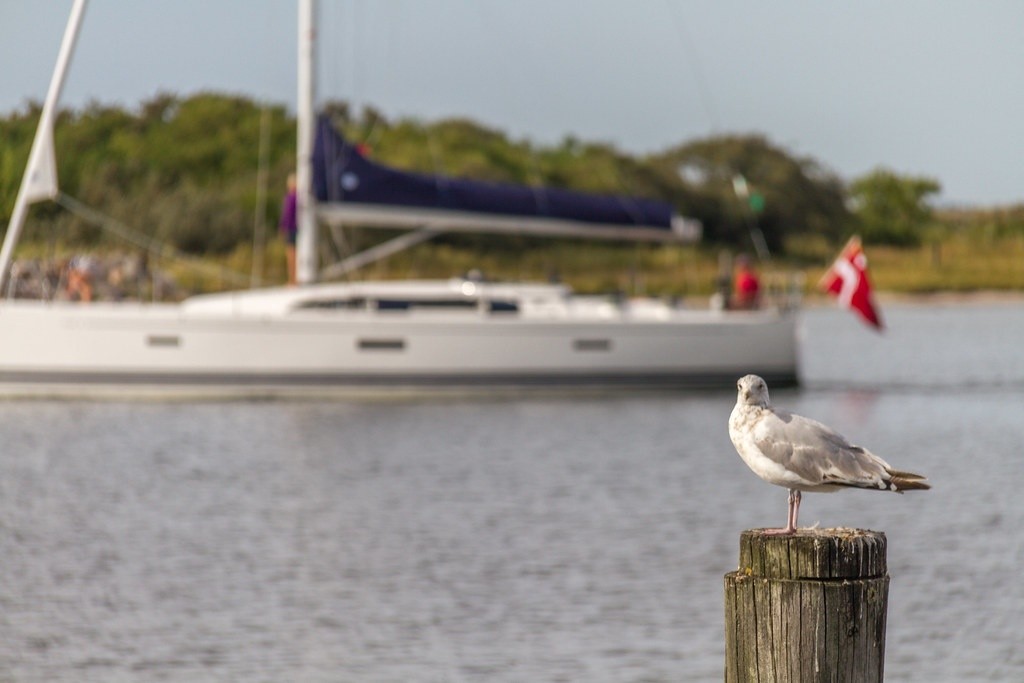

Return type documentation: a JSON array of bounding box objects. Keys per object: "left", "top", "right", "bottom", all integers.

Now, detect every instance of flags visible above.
[{"left": 829, "top": 240, "right": 884, "bottom": 332}]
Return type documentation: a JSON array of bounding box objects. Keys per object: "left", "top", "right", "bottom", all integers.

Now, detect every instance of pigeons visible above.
[{"left": 728, "top": 374, "right": 932, "bottom": 535}]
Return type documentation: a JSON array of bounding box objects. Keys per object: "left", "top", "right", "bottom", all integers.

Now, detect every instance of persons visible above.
[
  {"left": 280, "top": 172, "right": 296, "bottom": 283},
  {"left": 729, "top": 251, "right": 763, "bottom": 306}
]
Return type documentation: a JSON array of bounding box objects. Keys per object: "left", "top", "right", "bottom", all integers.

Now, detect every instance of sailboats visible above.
[{"left": 0, "top": 0, "right": 803, "bottom": 397}]
